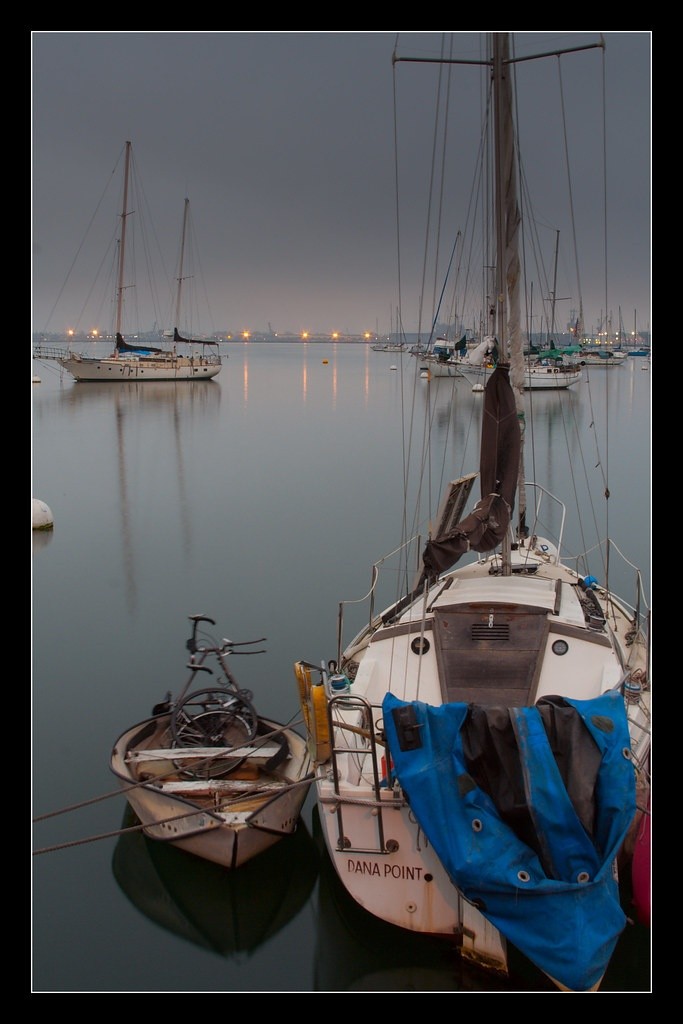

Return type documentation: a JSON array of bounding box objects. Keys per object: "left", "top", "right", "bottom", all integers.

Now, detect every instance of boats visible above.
[
  {"left": 107, "top": 800, "right": 324, "bottom": 956},
  {"left": 107, "top": 613, "right": 315, "bottom": 867}
]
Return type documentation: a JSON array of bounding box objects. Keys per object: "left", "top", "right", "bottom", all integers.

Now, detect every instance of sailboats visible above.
[
  {"left": 366, "top": 218, "right": 648, "bottom": 388},
  {"left": 31, "top": 138, "right": 223, "bottom": 381},
  {"left": 307, "top": 20, "right": 653, "bottom": 989}
]
